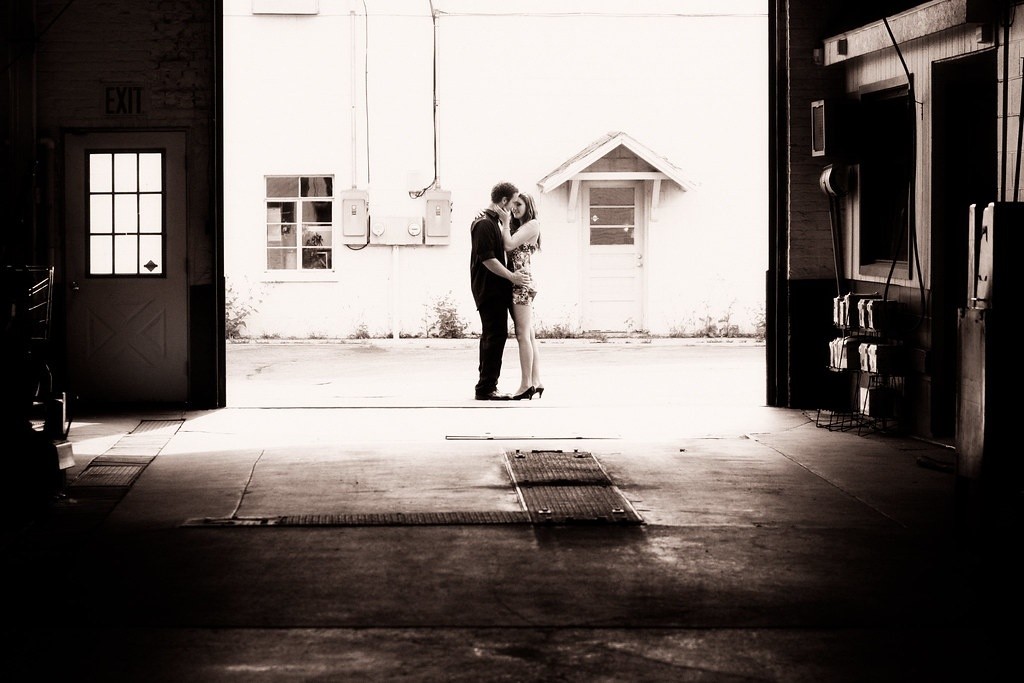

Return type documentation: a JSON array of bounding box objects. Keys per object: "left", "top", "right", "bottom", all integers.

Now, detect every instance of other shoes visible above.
[{"left": 475, "top": 389, "right": 513, "bottom": 400}]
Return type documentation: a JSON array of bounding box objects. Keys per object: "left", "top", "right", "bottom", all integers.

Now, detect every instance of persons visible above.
[
  {"left": 475, "top": 192, "right": 544, "bottom": 400},
  {"left": 470, "top": 182, "right": 530, "bottom": 400}
]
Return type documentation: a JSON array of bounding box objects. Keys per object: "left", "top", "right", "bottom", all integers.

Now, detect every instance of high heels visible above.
[
  {"left": 513, "top": 386, "right": 535, "bottom": 400},
  {"left": 535, "top": 382, "right": 544, "bottom": 398}
]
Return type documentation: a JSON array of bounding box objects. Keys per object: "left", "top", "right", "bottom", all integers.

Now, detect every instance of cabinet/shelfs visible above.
[{"left": 815, "top": 292, "right": 907, "bottom": 437}]
